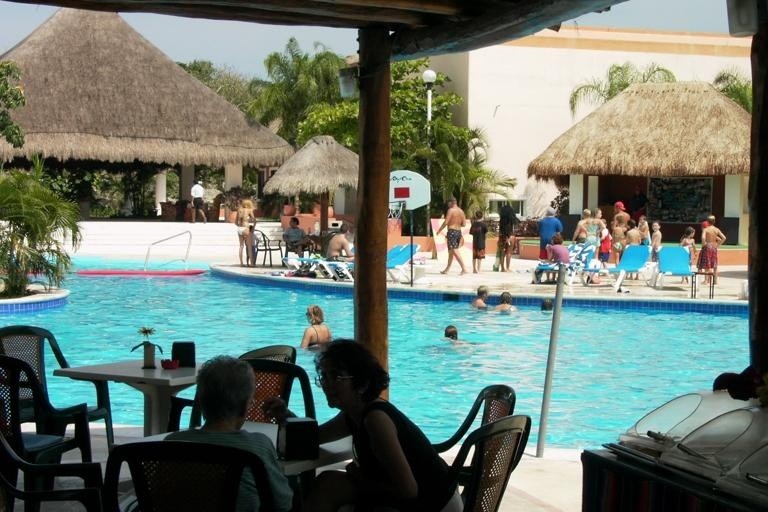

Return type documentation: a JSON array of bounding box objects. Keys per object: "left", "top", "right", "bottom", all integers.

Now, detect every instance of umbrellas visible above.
[{"left": 261, "top": 134, "right": 360, "bottom": 259}]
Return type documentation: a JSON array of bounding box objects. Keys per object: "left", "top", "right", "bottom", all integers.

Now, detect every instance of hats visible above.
[{"left": 615, "top": 201, "right": 626, "bottom": 210}]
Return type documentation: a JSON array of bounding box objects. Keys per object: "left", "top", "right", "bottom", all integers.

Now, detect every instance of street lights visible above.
[{"left": 419, "top": 69, "right": 437, "bottom": 237}]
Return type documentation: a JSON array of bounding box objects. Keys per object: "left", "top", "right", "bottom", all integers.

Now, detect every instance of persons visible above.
[
  {"left": 469, "top": 210, "right": 489, "bottom": 274},
  {"left": 435, "top": 198, "right": 469, "bottom": 276},
  {"left": 495, "top": 291, "right": 516, "bottom": 311},
  {"left": 149, "top": 355, "right": 294, "bottom": 511},
  {"left": 300, "top": 304, "right": 332, "bottom": 347},
  {"left": 541, "top": 299, "right": 554, "bottom": 310},
  {"left": 244, "top": 225, "right": 260, "bottom": 267},
  {"left": 627, "top": 183, "right": 651, "bottom": 222},
  {"left": 492, "top": 199, "right": 727, "bottom": 287},
  {"left": 259, "top": 338, "right": 465, "bottom": 512},
  {"left": 281, "top": 217, "right": 308, "bottom": 258},
  {"left": 189, "top": 179, "right": 208, "bottom": 224},
  {"left": 234, "top": 199, "right": 256, "bottom": 267},
  {"left": 326, "top": 223, "right": 355, "bottom": 262},
  {"left": 472, "top": 286, "right": 490, "bottom": 308},
  {"left": 445, "top": 324, "right": 460, "bottom": 344}
]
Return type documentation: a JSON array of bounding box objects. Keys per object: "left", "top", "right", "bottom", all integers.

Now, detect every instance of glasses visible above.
[{"left": 313, "top": 373, "right": 356, "bottom": 388}]
